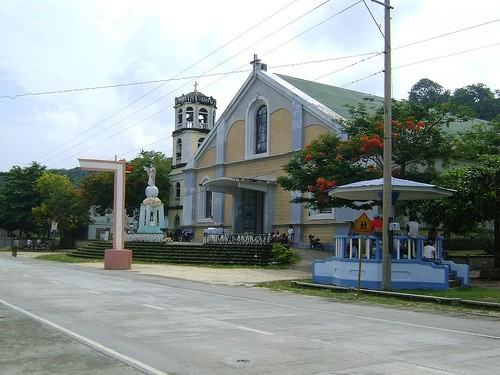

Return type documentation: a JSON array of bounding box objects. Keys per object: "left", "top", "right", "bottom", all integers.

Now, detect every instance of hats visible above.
[{"left": 373, "top": 214, "right": 379, "bottom": 218}]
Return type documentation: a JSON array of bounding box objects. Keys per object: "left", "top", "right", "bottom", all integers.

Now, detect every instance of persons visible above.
[
  {"left": 11, "top": 234, "right": 20, "bottom": 257},
  {"left": 401, "top": 215, "right": 419, "bottom": 249},
  {"left": 271, "top": 224, "right": 295, "bottom": 244},
  {"left": 368, "top": 214, "right": 383, "bottom": 259},
  {"left": 170, "top": 212, "right": 195, "bottom": 241},
  {"left": 423, "top": 240, "right": 436, "bottom": 259},
  {"left": 26, "top": 237, "right": 49, "bottom": 250},
  {"left": 142, "top": 160, "right": 157, "bottom": 186},
  {"left": 346, "top": 222, "right": 359, "bottom": 259}
]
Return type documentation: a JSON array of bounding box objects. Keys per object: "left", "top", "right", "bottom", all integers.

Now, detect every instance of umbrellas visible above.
[{"left": 324, "top": 177, "right": 457, "bottom": 235}]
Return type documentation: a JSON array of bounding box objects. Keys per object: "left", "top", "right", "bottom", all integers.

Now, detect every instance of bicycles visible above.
[{"left": 304, "top": 234, "right": 325, "bottom": 252}]
